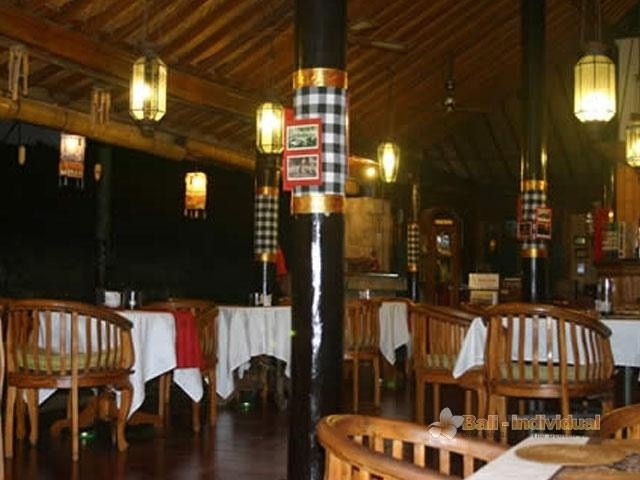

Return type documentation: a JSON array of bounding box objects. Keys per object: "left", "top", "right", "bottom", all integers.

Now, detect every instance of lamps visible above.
[
  {"left": 572, "top": 1, "right": 640, "bottom": 168},
  {"left": 377, "top": 93, "right": 402, "bottom": 185}
]
